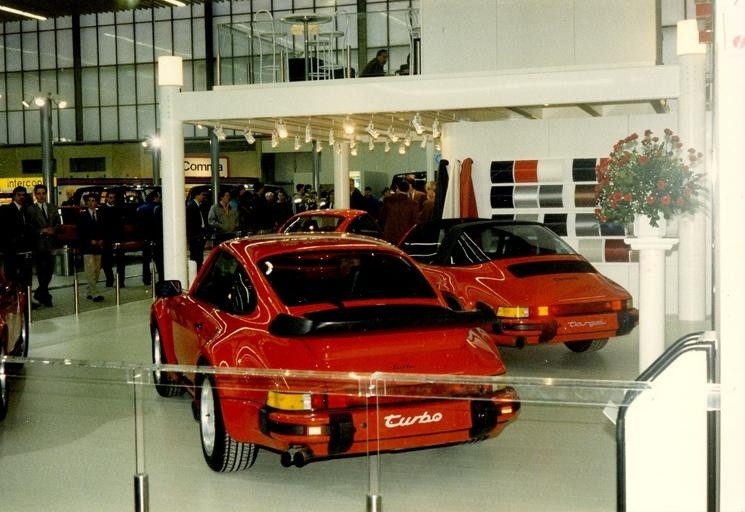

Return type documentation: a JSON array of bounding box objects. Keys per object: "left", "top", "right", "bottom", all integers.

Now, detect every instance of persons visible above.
[
  {"left": 358, "top": 49, "right": 388, "bottom": 77},
  {"left": 394, "top": 53, "right": 409, "bottom": 75}
]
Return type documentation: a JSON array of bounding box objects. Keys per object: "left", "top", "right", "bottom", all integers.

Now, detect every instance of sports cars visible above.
[
  {"left": 396, "top": 218, "right": 640, "bottom": 358},
  {"left": 146, "top": 230, "right": 519, "bottom": 475}
]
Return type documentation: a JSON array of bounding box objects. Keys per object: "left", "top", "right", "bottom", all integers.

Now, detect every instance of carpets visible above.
[{"left": 29, "top": 248, "right": 212, "bottom": 323}]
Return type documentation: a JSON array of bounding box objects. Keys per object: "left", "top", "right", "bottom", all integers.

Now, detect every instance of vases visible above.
[{"left": 632, "top": 211, "right": 668, "bottom": 239}]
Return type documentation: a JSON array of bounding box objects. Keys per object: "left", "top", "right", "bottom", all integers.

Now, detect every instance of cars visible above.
[
  {"left": 0, "top": 232, "right": 30, "bottom": 417},
  {"left": 57, "top": 183, "right": 161, "bottom": 255},
  {"left": 276, "top": 208, "right": 377, "bottom": 237}
]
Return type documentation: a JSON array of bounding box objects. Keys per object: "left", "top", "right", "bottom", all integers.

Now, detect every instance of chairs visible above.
[{"left": 250, "top": 4, "right": 422, "bottom": 86}]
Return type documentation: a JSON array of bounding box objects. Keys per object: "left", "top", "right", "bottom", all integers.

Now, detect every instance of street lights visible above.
[{"left": 23, "top": 91, "right": 65, "bottom": 206}]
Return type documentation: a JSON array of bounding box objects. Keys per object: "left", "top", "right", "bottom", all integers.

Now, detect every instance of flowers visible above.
[{"left": 591, "top": 128, "right": 712, "bottom": 229}]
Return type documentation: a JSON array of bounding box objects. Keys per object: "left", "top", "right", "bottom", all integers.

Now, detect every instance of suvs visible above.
[
  {"left": 246, "top": 184, "right": 292, "bottom": 203},
  {"left": 183, "top": 182, "right": 242, "bottom": 206}
]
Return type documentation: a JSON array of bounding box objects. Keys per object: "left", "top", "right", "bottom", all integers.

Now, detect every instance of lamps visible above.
[
  {"left": 19, "top": 88, "right": 68, "bottom": 110},
  {"left": 206, "top": 104, "right": 445, "bottom": 160}
]
